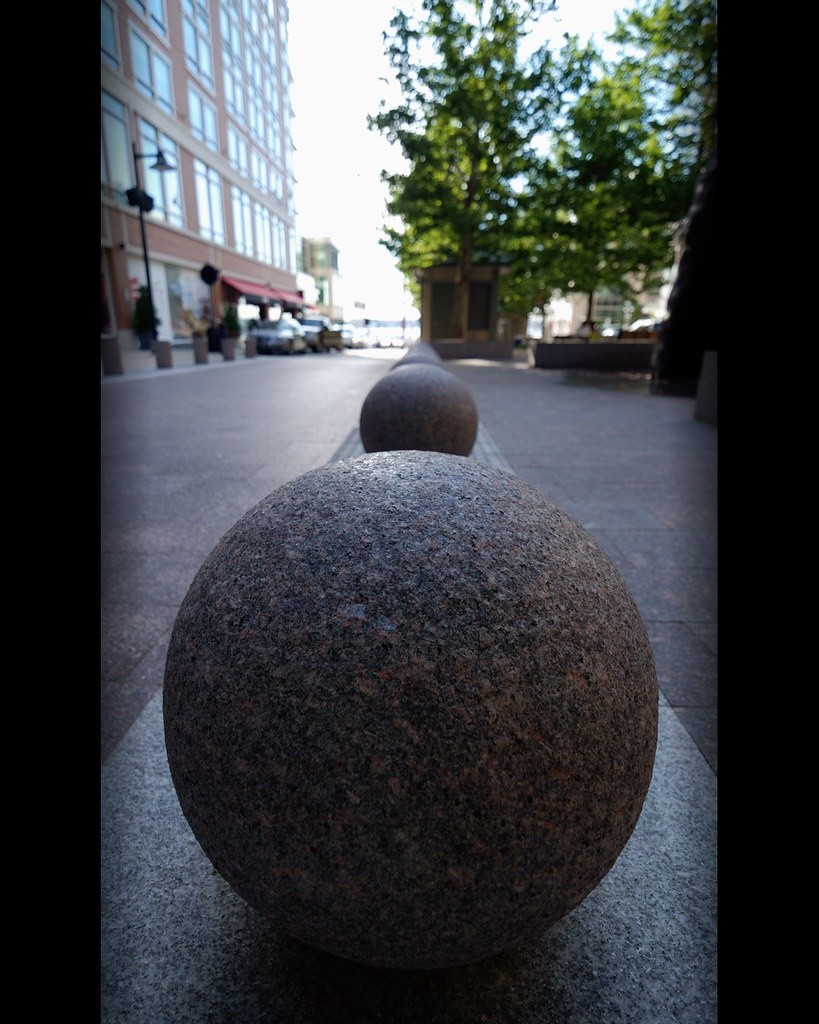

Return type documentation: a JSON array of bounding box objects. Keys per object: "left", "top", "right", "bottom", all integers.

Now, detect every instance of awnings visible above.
[{"left": 222, "top": 277, "right": 314, "bottom": 309}]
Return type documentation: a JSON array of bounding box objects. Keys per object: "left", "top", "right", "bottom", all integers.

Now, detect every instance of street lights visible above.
[{"left": 130, "top": 141, "right": 177, "bottom": 341}]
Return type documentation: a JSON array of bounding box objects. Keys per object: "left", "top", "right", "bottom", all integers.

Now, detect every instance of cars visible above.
[
  {"left": 296, "top": 313, "right": 420, "bottom": 355},
  {"left": 254, "top": 315, "right": 308, "bottom": 357},
  {"left": 627, "top": 317, "right": 670, "bottom": 339}
]
